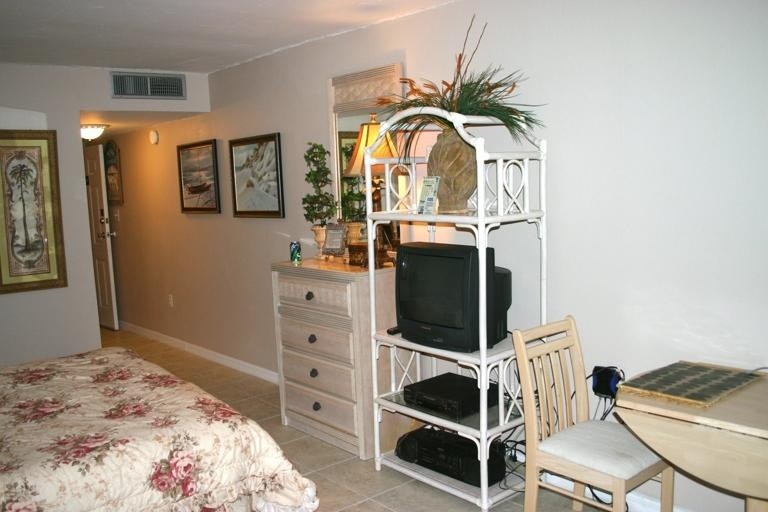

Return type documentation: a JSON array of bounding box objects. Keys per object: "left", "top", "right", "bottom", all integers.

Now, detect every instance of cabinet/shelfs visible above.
[
  {"left": 269, "top": 253, "right": 418, "bottom": 461},
  {"left": 364, "top": 108, "right": 548, "bottom": 512}
]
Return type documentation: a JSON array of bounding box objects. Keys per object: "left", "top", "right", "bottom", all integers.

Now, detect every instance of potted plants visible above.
[
  {"left": 302, "top": 142, "right": 338, "bottom": 259},
  {"left": 342, "top": 143, "right": 365, "bottom": 245},
  {"left": 374, "top": 15, "right": 545, "bottom": 215}
]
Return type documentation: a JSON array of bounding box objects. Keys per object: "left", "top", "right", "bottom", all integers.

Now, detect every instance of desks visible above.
[{"left": 615, "top": 363, "right": 768, "bottom": 512}]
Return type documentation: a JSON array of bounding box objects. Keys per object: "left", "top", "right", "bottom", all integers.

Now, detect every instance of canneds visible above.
[{"left": 290, "top": 240, "right": 301, "bottom": 262}]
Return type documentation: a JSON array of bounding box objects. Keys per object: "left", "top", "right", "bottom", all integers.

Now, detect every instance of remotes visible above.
[{"left": 387, "top": 326, "right": 402, "bottom": 335}]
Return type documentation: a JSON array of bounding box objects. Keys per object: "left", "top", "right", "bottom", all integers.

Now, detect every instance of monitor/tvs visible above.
[{"left": 395, "top": 242, "right": 513, "bottom": 354}]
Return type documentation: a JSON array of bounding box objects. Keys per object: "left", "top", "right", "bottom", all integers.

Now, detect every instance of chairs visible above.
[{"left": 513, "top": 314, "right": 676, "bottom": 512}]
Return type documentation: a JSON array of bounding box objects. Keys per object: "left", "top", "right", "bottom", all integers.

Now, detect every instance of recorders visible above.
[{"left": 395, "top": 423, "right": 506, "bottom": 488}]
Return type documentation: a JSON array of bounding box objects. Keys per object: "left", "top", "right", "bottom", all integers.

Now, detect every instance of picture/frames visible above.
[
  {"left": 0, "top": 129, "right": 67, "bottom": 294},
  {"left": 177, "top": 139, "right": 221, "bottom": 214},
  {"left": 339, "top": 132, "right": 367, "bottom": 222},
  {"left": 229, "top": 132, "right": 284, "bottom": 217},
  {"left": 104, "top": 141, "right": 124, "bottom": 204}
]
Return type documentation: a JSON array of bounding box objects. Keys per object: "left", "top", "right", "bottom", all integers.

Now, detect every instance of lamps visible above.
[
  {"left": 346, "top": 113, "right": 407, "bottom": 213},
  {"left": 80, "top": 124, "right": 110, "bottom": 141}
]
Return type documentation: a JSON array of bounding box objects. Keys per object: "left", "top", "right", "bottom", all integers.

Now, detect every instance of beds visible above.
[{"left": 0, "top": 346, "right": 320, "bottom": 512}]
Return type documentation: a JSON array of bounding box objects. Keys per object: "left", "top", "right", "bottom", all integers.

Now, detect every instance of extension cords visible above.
[{"left": 505, "top": 455, "right": 545, "bottom": 478}]
